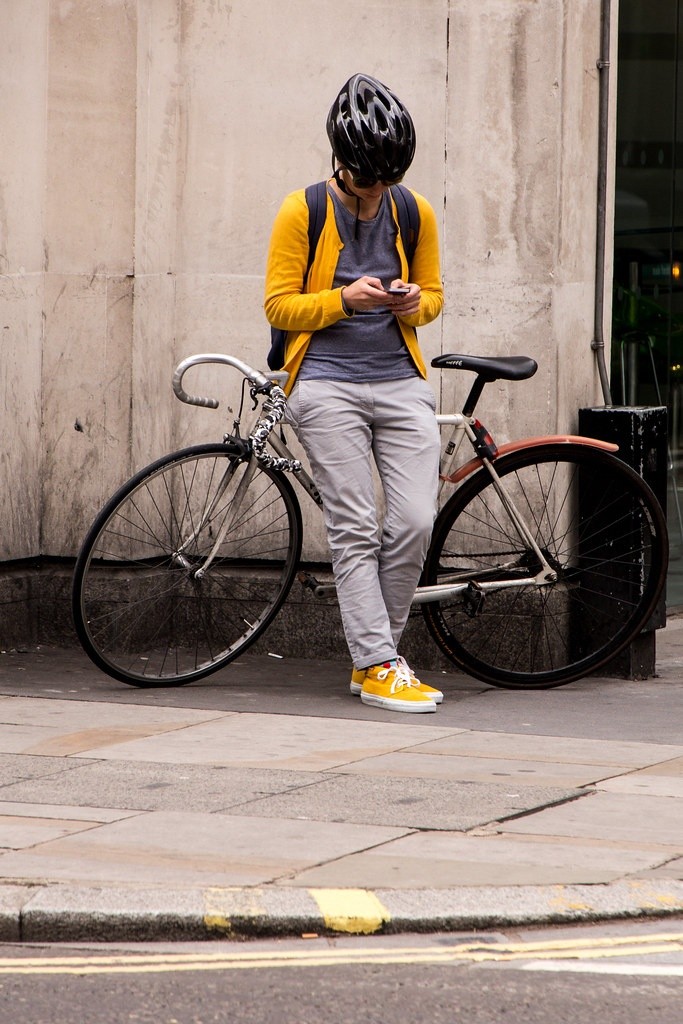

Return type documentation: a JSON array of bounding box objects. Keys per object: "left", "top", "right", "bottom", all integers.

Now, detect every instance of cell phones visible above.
[{"left": 386, "top": 288, "right": 411, "bottom": 294}]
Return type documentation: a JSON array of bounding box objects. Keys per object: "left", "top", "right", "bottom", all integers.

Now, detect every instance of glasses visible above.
[{"left": 351, "top": 171, "right": 405, "bottom": 189}]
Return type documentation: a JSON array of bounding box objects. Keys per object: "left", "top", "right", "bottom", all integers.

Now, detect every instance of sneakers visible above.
[
  {"left": 349, "top": 655, "right": 444, "bottom": 704},
  {"left": 361, "top": 665, "right": 437, "bottom": 713}
]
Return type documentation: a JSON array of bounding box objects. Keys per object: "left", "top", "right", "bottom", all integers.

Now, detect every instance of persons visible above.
[{"left": 264, "top": 72, "right": 445, "bottom": 713}]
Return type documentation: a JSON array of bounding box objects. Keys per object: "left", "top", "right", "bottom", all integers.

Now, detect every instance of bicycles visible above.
[{"left": 71, "top": 352, "right": 670, "bottom": 689}]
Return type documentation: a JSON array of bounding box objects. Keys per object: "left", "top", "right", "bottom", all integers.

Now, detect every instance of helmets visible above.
[{"left": 326, "top": 74, "right": 416, "bottom": 178}]
